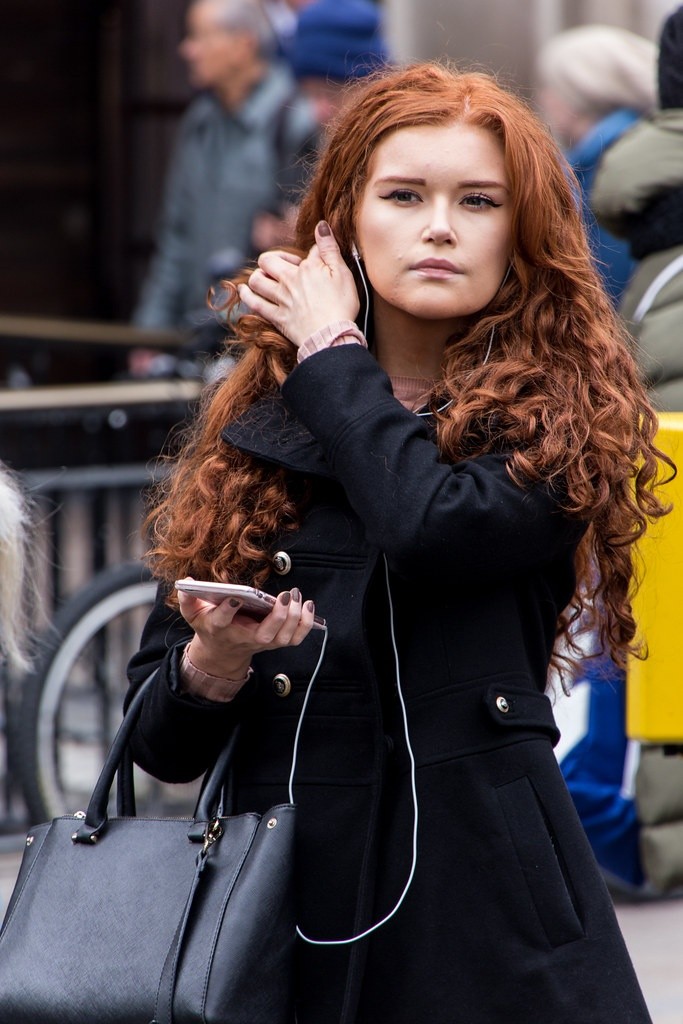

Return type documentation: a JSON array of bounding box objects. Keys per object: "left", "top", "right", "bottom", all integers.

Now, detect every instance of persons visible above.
[
  {"left": 584, "top": 4, "right": 682, "bottom": 903},
  {"left": 129, "top": 2, "right": 385, "bottom": 462},
  {"left": 532, "top": 25, "right": 660, "bottom": 314},
  {"left": 122, "top": 59, "right": 675, "bottom": 1024}
]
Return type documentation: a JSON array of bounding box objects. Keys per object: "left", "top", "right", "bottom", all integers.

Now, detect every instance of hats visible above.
[
  {"left": 544, "top": 23, "right": 655, "bottom": 114},
  {"left": 657, "top": 5, "right": 683, "bottom": 111},
  {"left": 268, "top": 0, "right": 389, "bottom": 80}
]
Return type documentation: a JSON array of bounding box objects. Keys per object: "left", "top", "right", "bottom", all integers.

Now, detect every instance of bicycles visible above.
[{"left": 8, "top": 562, "right": 224, "bottom": 830}]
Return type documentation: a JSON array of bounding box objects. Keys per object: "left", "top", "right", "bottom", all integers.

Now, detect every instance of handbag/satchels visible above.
[{"left": 0, "top": 658, "right": 294, "bottom": 1020}]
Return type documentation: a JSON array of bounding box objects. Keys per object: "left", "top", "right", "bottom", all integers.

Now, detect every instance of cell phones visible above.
[{"left": 174, "top": 580, "right": 327, "bottom": 629}]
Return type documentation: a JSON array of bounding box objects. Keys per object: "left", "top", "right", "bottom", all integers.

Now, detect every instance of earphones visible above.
[{"left": 349, "top": 242, "right": 360, "bottom": 256}]
